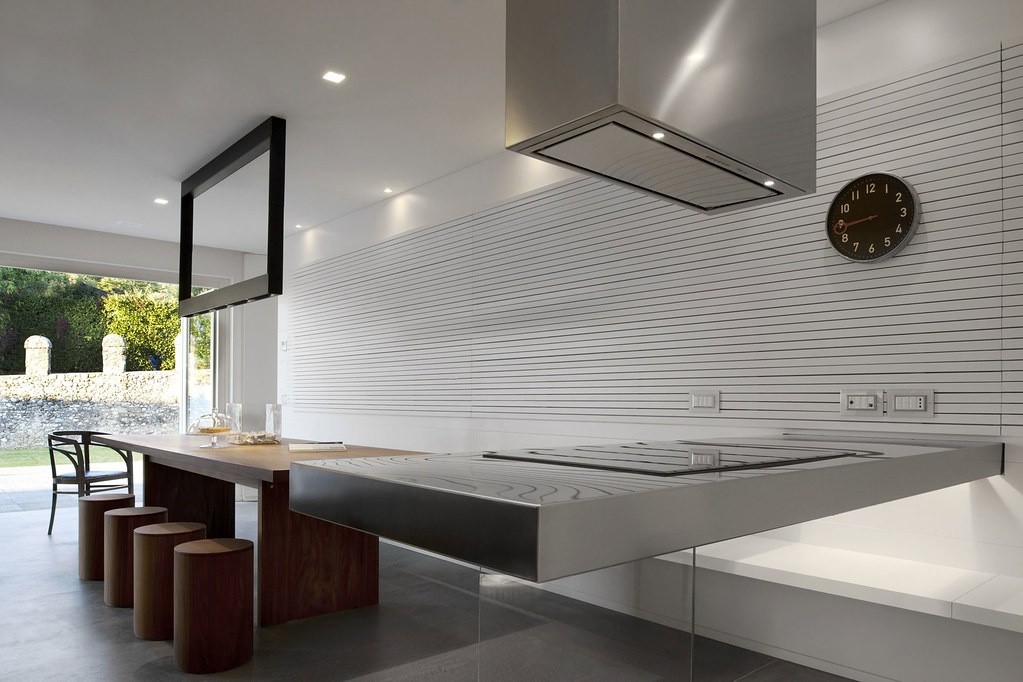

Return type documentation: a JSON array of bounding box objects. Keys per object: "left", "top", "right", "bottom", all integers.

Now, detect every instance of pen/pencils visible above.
[{"left": 307, "top": 441, "right": 343, "bottom": 444}]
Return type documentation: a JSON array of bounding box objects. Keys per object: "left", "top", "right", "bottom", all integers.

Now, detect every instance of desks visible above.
[{"left": 90, "top": 432, "right": 438, "bottom": 628}]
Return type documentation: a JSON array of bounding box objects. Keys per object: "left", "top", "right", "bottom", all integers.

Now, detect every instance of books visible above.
[{"left": 288, "top": 442, "right": 348, "bottom": 452}]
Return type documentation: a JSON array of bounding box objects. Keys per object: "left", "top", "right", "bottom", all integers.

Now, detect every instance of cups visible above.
[
  {"left": 226, "top": 403, "right": 242, "bottom": 434},
  {"left": 265, "top": 404, "right": 282, "bottom": 439}
]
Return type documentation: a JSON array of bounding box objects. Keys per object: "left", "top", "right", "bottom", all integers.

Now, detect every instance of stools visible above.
[
  {"left": 133, "top": 523, "right": 206, "bottom": 642},
  {"left": 104, "top": 506, "right": 168, "bottom": 608},
  {"left": 78, "top": 492, "right": 138, "bottom": 580},
  {"left": 172, "top": 539, "right": 254, "bottom": 675}
]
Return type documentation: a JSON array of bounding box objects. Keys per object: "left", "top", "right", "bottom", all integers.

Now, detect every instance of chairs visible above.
[{"left": 46, "top": 429, "right": 133, "bottom": 537}]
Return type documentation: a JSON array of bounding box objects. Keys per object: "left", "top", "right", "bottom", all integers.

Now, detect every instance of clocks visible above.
[{"left": 825, "top": 171, "right": 922, "bottom": 264}]
